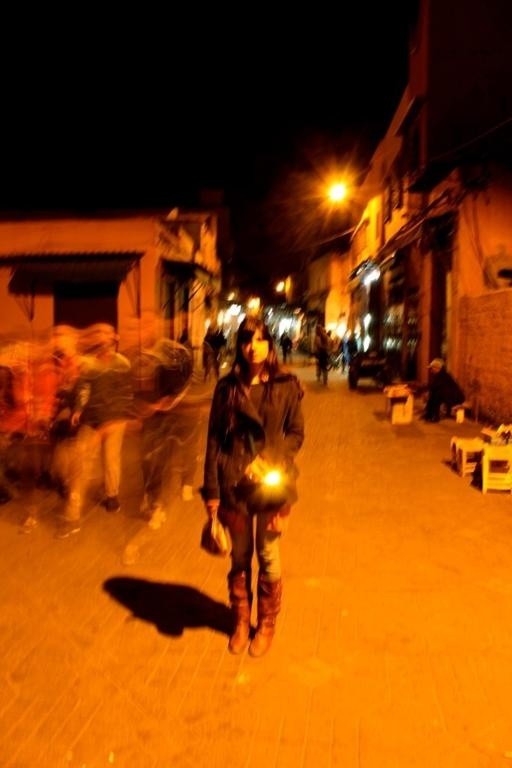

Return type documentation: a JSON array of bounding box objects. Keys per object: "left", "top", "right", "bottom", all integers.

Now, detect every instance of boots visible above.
[
  {"left": 248, "top": 576, "right": 282, "bottom": 657},
  {"left": 227, "top": 572, "right": 253, "bottom": 656}
]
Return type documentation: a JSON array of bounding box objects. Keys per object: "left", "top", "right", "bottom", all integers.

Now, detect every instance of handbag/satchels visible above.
[{"left": 201, "top": 506, "right": 230, "bottom": 555}]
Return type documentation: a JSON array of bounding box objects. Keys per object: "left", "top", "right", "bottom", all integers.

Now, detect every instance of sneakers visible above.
[{"left": 19, "top": 485, "right": 192, "bottom": 541}]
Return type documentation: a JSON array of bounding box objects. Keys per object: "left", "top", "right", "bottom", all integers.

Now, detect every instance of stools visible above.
[{"left": 452, "top": 405, "right": 464, "bottom": 423}]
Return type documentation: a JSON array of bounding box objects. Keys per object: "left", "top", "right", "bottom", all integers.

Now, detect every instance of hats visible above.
[{"left": 425, "top": 359, "right": 442, "bottom": 368}]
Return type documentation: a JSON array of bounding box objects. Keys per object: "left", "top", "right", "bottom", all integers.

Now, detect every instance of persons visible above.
[
  {"left": 201, "top": 323, "right": 358, "bottom": 388},
  {"left": 56, "top": 323, "right": 134, "bottom": 538},
  {"left": 121, "top": 307, "right": 194, "bottom": 527},
  {"left": 418, "top": 360, "right": 465, "bottom": 422},
  {"left": 197, "top": 321, "right": 304, "bottom": 661}
]
